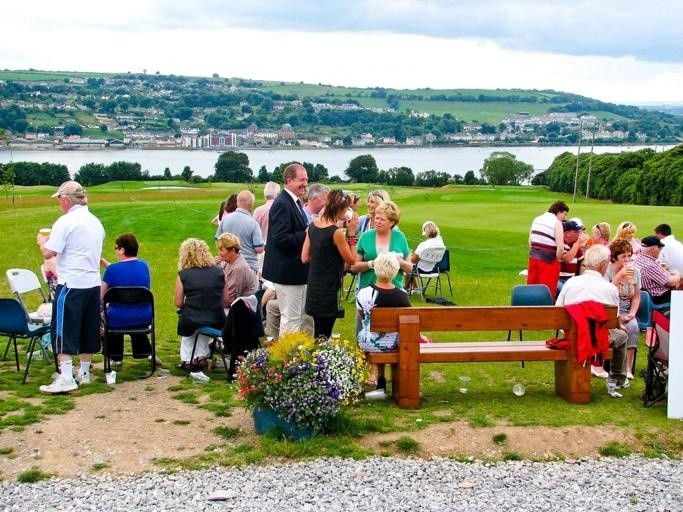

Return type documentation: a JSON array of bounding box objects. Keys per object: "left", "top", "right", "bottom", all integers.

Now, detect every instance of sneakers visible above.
[
  {"left": 591, "top": 365, "right": 634, "bottom": 389},
  {"left": 76, "top": 369, "right": 91, "bottom": 385},
  {"left": 183, "top": 360, "right": 208, "bottom": 372},
  {"left": 376, "top": 374, "right": 387, "bottom": 392},
  {"left": 39, "top": 373, "right": 78, "bottom": 393},
  {"left": 368, "top": 379, "right": 377, "bottom": 387}
]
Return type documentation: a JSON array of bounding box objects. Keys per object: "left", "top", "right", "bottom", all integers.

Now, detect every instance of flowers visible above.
[{"left": 231, "top": 328, "right": 373, "bottom": 431}]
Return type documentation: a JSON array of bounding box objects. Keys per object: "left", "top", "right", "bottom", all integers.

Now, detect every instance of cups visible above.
[
  {"left": 365, "top": 389, "right": 386, "bottom": 401},
  {"left": 660, "top": 260, "right": 668, "bottom": 269},
  {"left": 624, "top": 261, "right": 636, "bottom": 278},
  {"left": 606, "top": 378, "right": 617, "bottom": 394},
  {"left": 39, "top": 229, "right": 51, "bottom": 238},
  {"left": 332, "top": 333, "right": 342, "bottom": 340},
  {"left": 459, "top": 377, "right": 470, "bottom": 393},
  {"left": 105, "top": 372, "right": 117, "bottom": 383},
  {"left": 27, "top": 350, "right": 43, "bottom": 361}
]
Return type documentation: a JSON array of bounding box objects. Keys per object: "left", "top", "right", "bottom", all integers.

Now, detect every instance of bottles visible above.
[{"left": 513, "top": 384, "right": 525, "bottom": 396}]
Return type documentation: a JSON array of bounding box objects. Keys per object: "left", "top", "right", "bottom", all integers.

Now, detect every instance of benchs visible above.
[{"left": 371, "top": 302, "right": 620, "bottom": 411}]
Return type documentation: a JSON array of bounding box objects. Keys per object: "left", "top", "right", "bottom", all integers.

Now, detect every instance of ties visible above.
[{"left": 297, "top": 199, "right": 309, "bottom": 226}]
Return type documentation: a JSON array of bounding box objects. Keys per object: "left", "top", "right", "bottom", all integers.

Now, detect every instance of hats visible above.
[
  {"left": 564, "top": 221, "right": 586, "bottom": 232},
  {"left": 51, "top": 181, "right": 83, "bottom": 198},
  {"left": 641, "top": 236, "right": 665, "bottom": 247}
]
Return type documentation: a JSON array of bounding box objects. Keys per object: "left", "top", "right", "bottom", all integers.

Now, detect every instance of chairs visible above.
[
  {"left": 506, "top": 282, "right": 561, "bottom": 369},
  {"left": 406, "top": 249, "right": 452, "bottom": 304},
  {"left": 3, "top": 268, "right": 261, "bottom": 387},
  {"left": 631, "top": 290, "right": 671, "bottom": 408}
]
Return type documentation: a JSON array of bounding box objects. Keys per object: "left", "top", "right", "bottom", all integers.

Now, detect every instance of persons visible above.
[
  {"left": 174, "top": 163, "right": 412, "bottom": 394},
  {"left": 41, "top": 249, "right": 105, "bottom": 341},
  {"left": 523, "top": 196, "right": 683, "bottom": 393},
  {"left": 404, "top": 222, "right": 444, "bottom": 290},
  {"left": 94, "top": 230, "right": 164, "bottom": 368},
  {"left": 34, "top": 181, "right": 106, "bottom": 395}
]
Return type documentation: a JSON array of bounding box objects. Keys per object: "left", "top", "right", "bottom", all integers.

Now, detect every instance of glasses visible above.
[
  {"left": 373, "top": 191, "right": 384, "bottom": 200},
  {"left": 115, "top": 246, "right": 118, "bottom": 250}
]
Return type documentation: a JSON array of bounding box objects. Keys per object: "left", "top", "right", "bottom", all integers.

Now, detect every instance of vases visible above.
[{"left": 253, "top": 404, "right": 324, "bottom": 442}]
